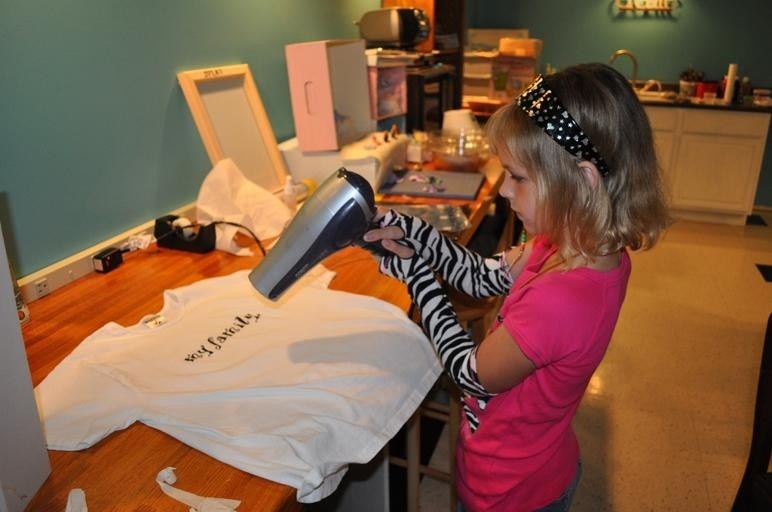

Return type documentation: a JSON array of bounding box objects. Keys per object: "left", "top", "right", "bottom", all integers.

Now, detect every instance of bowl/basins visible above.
[{"left": 428, "top": 133, "right": 494, "bottom": 172}]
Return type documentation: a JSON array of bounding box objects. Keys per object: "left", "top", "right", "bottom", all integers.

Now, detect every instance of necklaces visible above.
[{"left": 503, "top": 229, "right": 614, "bottom": 296}]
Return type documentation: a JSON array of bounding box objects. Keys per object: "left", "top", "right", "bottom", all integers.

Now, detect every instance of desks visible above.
[{"left": 18, "top": 135, "right": 522, "bottom": 511}]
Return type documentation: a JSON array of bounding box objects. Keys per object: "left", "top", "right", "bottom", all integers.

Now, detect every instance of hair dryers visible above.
[{"left": 248, "top": 168, "right": 388, "bottom": 303}]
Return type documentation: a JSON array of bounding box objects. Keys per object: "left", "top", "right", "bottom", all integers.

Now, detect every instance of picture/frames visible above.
[{"left": 176, "top": 63, "right": 293, "bottom": 196}]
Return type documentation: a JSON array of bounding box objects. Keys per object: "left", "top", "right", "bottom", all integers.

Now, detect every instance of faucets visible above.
[{"left": 608, "top": 47, "right": 638, "bottom": 89}]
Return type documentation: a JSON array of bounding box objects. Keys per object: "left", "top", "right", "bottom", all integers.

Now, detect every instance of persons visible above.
[{"left": 363, "top": 63, "right": 679, "bottom": 512}]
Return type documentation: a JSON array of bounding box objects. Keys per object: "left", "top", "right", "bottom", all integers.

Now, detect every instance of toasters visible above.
[{"left": 361, "top": 10, "right": 430, "bottom": 49}]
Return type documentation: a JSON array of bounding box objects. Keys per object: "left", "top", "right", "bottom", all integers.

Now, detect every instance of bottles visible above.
[{"left": 741, "top": 77, "right": 754, "bottom": 104}]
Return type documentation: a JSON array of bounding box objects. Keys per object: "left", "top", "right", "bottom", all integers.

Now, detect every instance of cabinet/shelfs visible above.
[{"left": 637, "top": 106, "right": 769, "bottom": 226}]
